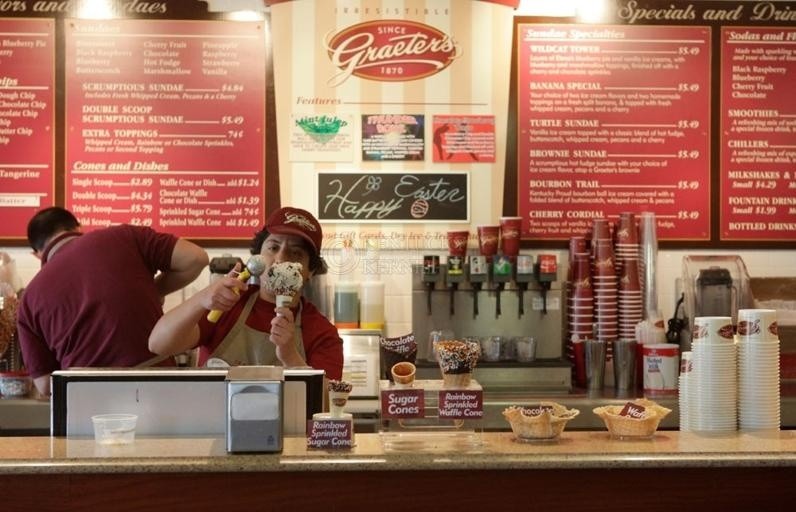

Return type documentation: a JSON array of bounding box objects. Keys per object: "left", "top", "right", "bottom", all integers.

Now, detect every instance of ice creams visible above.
[
  {"left": 328, "top": 379, "right": 353, "bottom": 417},
  {"left": 267, "top": 262, "right": 304, "bottom": 316}
]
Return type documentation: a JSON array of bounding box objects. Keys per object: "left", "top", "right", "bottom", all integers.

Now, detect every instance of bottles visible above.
[
  {"left": 338, "top": 239, "right": 356, "bottom": 281},
  {"left": 363, "top": 238, "right": 381, "bottom": 281}
]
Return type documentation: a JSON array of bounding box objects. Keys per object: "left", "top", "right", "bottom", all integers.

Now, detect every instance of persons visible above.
[
  {"left": 19, "top": 206, "right": 209, "bottom": 396},
  {"left": 149, "top": 204, "right": 344, "bottom": 420}
]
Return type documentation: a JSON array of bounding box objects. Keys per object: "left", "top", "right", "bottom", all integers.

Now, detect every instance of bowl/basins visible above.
[
  {"left": 601, "top": 419, "right": 660, "bottom": 440},
  {"left": 689, "top": 316, "right": 737, "bottom": 438},
  {"left": 737, "top": 308, "right": 779, "bottom": 431},
  {"left": 511, "top": 420, "right": 565, "bottom": 442},
  {"left": 90, "top": 414, "right": 138, "bottom": 443},
  {"left": 677, "top": 350, "right": 694, "bottom": 430},
  {"left": 0, "top": 372, "right": 33, "bottom": 399}
]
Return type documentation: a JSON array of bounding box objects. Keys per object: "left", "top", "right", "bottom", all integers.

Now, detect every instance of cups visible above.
[
  {"left": 611, "top": 338, "right": 638, "bottom": 393},
  {"left": 616, "top": 261, "right": 643, "bottom": 340},
  {"left": 425, "top": 328, "right": 455, "bottom": 363},
  {"left": 638, "top": 211, "right": 660, "bottom": 318},
  {"left": 589, "top": 218, "right": 610, "bottom": 271},
  {"left": 565, "top": 236, "right": 587, "bottom": 325},
  {"left": 613, "top": 211, "right": 640, "bottom": 279},
  {"left": 500, "top": 216, "right": 523, "bottom": 258},
  {"left": 477, "top": 225, "right": 498, "bottom": 264},
  {"left": 330, "top": 282, "right": 387, "bottom": 332},
  {"left": 465, "top": 335, "right": 538, "bottom": 363},
  {"left": 570, "top": 338, "right": 588, "bottom": 389},
  {"left": 585, "top": 341, "right": 609, "bottom": 389},
  {"left": 447, "top": 231, "right": 473, "bottom": 263},
  {"left": 591, "top": 224, "right": 617, "bottom": 361},
  {"left": 566, "top": 253, "right": 594, "bottom": 361}
]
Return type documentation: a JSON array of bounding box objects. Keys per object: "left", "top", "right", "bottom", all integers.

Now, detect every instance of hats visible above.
[{"left": 263, "top": 206, "right": 323, "bottom": 257}]
